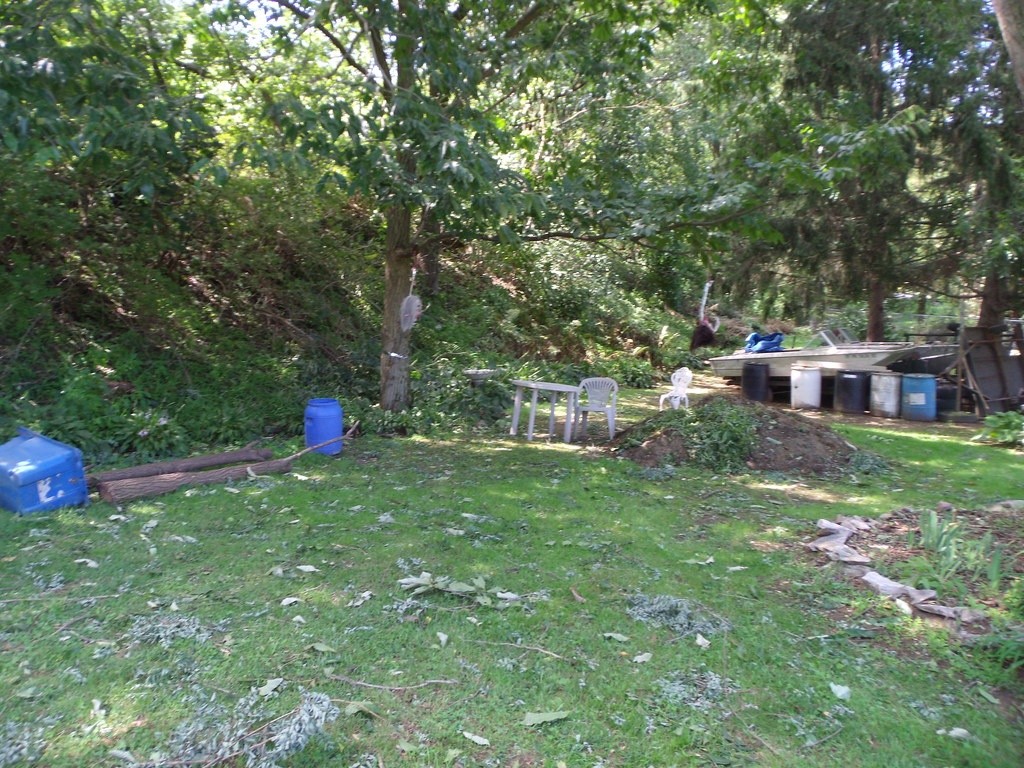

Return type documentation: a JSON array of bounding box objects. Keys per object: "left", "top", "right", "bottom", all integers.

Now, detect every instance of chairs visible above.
[
  {"left": 574, "top": 377, "right": 619, "bottom": 440},
  {"left": 659, "top": 366, "right": 694, "bottom": 411}
]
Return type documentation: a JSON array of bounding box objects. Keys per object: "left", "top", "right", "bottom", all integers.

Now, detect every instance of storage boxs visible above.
[{"left": 0, "top": 426, "right": 90, "bottom": 517}]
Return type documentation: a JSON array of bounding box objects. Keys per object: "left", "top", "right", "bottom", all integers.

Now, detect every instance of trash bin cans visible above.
[
  {"left": 868, "top": 370, "right": 904, "bottom": 418},
  {"left": 740, "top": 360, "right": 770, "bottom": 403},
  {"left": 899, "top": 373, "right": 937, "bottom": 421},
  {"left": 833, "top": 367, "right": 869, "bottom": 414},
  {"left": 790, "top": 364, "right": 822, "bottom": 410}
]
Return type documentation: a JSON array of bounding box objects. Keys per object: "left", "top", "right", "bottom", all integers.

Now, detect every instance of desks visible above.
[{"left": 510, "top": 380, "right": 584, "bottom": 443}]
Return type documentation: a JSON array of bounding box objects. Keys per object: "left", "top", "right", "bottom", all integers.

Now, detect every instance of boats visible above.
[{"left": 706, "top": 327, "right": 961, "bottom": 393}]
